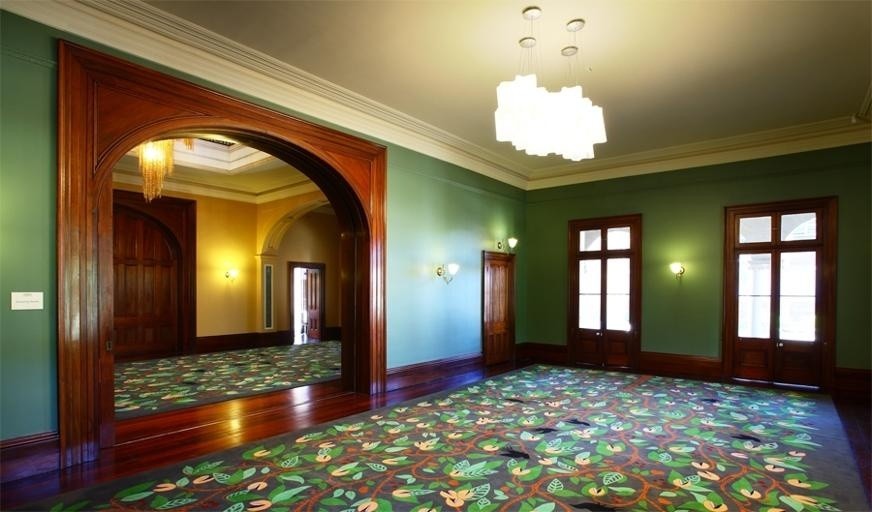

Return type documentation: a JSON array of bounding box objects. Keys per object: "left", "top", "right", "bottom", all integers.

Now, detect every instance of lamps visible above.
[
  {"left": 498, "top": 237, "right": 518, "bottom": 249},
  {"left": 494, "top": 6, "right": 607, "bottom": 162},
  {"left": 671, "top": 263, "right": 684, "bottom": 279},
  {"left": 437, "top": 263, "right": 457, "bottom": 284},
  {"left": 225, "top": 270, "right": 236, "bottom": 278},
  {"left": 136, "top": 137, "right": 191, "bottom": 203}
]
